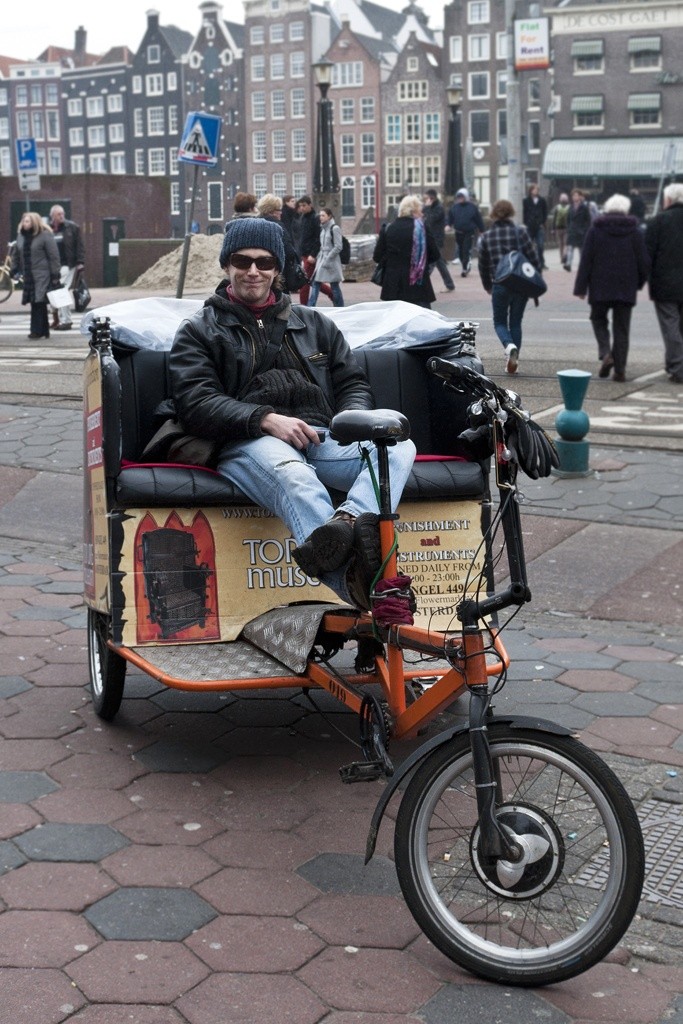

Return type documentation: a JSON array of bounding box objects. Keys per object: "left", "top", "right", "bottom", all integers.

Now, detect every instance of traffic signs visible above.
[{"left": 14, "top": 136, "right": 43, "bottom": 193}]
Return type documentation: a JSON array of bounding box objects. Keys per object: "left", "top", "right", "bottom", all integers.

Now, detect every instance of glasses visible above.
[
  {"left": 227, "top": 253, "right": 278, "bottom": 271},
  {"left": 275, "top": 208, "right": 282, "bottom": 213}
]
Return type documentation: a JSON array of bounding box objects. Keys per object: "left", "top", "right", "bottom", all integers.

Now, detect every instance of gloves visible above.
[{"left": 503, "top": 409, "right": 560, "bottom": 479}]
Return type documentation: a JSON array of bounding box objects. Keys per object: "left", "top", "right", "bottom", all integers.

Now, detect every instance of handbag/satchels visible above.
[
  {"left": 330, "top": 224, "right": 351, "bottom": 265},
  {"left": 283, "top": 262, "right": 308, "bottom": 294},
  {"left": 492, "top": 223, "right": 547, "bottom": 298},
  {"left": 48, "top": 281, "right": 74, "bottom": 309},
  {"left": 371, "top": 222, "right": 391, "bottom": 287},
  {"left": 143, "top": 417, "right": 220, "bottom": 470},
  {"left": 73, "top": 270, "right": 91, "bottom": 312}
]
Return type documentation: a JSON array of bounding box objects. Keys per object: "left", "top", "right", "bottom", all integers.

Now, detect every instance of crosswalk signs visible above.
[{"left": 176, "top": 111, "right": 223, "bottom": 170}]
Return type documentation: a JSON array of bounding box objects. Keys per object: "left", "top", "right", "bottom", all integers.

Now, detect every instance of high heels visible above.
[{"left": 28, "top": 331, "right": 50, "bottom": 338}]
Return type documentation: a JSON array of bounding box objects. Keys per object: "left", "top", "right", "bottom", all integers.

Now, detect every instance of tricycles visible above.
[{"left": 80, "top": 315, "right": 648, "bottom": 987}]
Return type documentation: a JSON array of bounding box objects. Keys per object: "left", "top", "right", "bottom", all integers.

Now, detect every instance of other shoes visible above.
[
  {"left": 462, "top": 266, "right": 471, "bottom": 277},
  {"left": 563, "top": 265, "right": 571, "bottom": 272},
  {"left": 50, "top": 321, "right": 72, "bottom": 329},
  {"left": 669, "top": 375, "right": 683, "bottom": 384},
  {"left": 452, "top": 258, "right": 460, "bottom": 264},
  {"left": 353, "top": 512, "right": 418, "bottom": 616},
  {"left": 504, "top": 344, "right": 519, "bottom": 373},
  {"left": 599, "top": 354, "right": 614, "bottom": 378},
  {"left": 287, "top": 518, "right": 357, "bottom": 578},
  {"left": 612, "top": 372, "right": 626, "bottom": 382},
  {"left": 440, "top": 288, "right": 452, "bottom": 293}
]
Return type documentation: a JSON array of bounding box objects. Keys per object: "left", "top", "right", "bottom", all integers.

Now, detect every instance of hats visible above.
[
  {"left": 559, "top": 194, "right": 567, "bottom": 202},
  {"left": 457, "top": 188, "right": 469, "bottom": 202},
  {"left": 220, "top": 217, "right": 285, "bottom": 276}
]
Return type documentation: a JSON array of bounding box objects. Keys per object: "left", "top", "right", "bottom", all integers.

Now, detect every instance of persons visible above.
[
  {"left": 445, "top": 187, "right": 483, "bottom": 276},
  {"left": 643, "top": 182, "right": 683, "bottom": 383},
  {"left": 478, "top": 198, "right": 541, "bottom": 373},
  {"left": 572, "top": 193, "right": 647, "bottom": 382},
  {"left": 254, "top": 193, "right": 302, "bottom": 303},
  {"left": 373, "top": 196, "right": 442, "bottom": 310},
  {"left": 43, "top": 204, "right": 85, "bottom": 331},
  {"left": 280, "top": 194, "right": 302, "bottom": 295},
  {"left": 167, "top": 213, "right": 417, "bottom": 613},
  {"left": 306, "top": 208, "right": 345, "bottom": 307},
  {"left": 299, "top": 196, "right": 333, "bottom": 306},
  {"left": 231, "top": 192, "right": 261, "bottom": 218},
  {"left": 563, "top": 191, "right": 592, "bottom": 271},
  {"left": 8, "top": 211, "right": 61, "bottom": 340},
  {"left": 550, "top": 192, "right": 571, "bottom": 264},
  {"left": 583, "top": 192, "right": 599, "bottom": 222},
  {"left": 523, "top": 183, "right": 548, "bottom": 269},
  {"left": 419, "top": 189, "right": 456, "bottom": 294},
  {"left": 627, "top": 189, "right": 647, "bottom": 228}
]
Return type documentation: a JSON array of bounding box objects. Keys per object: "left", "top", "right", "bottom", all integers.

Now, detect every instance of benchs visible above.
[{"left": 112, "top": 342, "right": 486, "bottom": 509}]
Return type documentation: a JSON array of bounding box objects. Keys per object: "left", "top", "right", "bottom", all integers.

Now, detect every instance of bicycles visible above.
[{"left": 0, "top": 240, "right": 25, "bottom": 304}]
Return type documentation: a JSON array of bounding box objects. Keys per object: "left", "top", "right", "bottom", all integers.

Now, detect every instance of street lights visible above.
[
  {"left": 307, "top": 52, "right": 344, "bottom": 228},
  {"left": 441, "top": 81, "right": 465, "bottom": 203}
]
